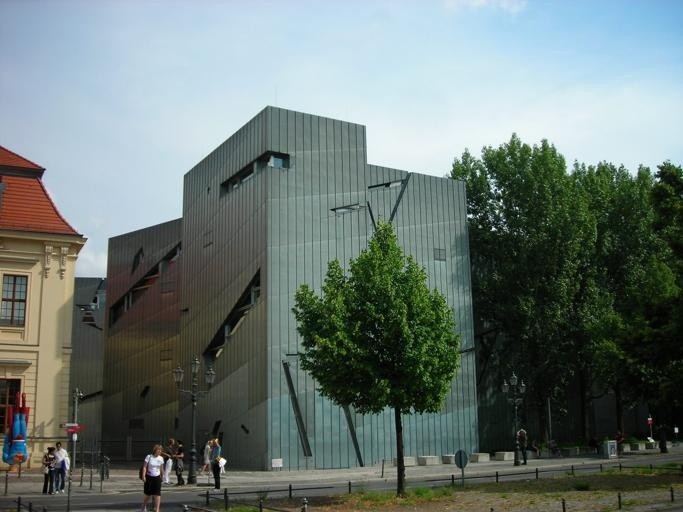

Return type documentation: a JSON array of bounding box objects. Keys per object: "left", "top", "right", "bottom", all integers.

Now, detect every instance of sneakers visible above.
[
  {"left": 49, "top": 490, "right": 65, "bottom": 495},
  {"left": 163, "top": 481, "right": 184, "bottom": 486}
]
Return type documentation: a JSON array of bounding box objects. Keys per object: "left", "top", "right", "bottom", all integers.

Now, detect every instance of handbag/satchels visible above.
[
  {"left": 40, "top": 463, "right": 50, "bottom": 475},
  {"left": 218, "top": 457, "right": 226, "bottom": 468},
  {"left": 139, "top": 464, "right": 148, "bottom": 479}
]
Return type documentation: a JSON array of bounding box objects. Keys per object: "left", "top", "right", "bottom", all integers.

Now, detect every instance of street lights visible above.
[
  {"left": 172, "top": 354, "right": 216, "bottom": 486},
  {"left": 500, "top": 370, "right": 527, "bottom": 468}
]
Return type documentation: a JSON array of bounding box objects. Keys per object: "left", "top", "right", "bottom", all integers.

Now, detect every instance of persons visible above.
[
  {"left": 209, "top": 437, "right": 222, "bottom": 491},
  {"left": 98, "top": 452, "right": 112, "bottom": 481},
  {"left": 141, "top": 443, "right": 166, "bottom": 512},
  {"left": 53, "top": 441, "right": 70, "bottom": 494},
  {"left": 2, "top": 390, "right": 31, "bottom": 473},
  {"left": 530, "top": 438, "right": 543, "bottom": 458},
  {"left": 159, "top": 437, "right": 176, "bottom": 485},
  {"left": 198, "top": 440, "right": 214, "bottom": 477},
  {"left": 587, "top": 431, "right": 603, "bottom": 454},
  {"left": 171, "top": 438, "right": 186, "bottom": 488},
  {"left": 516, "top": 424, "right": 528, "bottom": 466},
  {"left": 40, "top": 446, "right": 58, "bottom": 496},
  {"left": 656, "top": 424, "right": 670, "bottom": 454},
  {"left": 613, "top": 428, "right": 626, "bottom": 458}
]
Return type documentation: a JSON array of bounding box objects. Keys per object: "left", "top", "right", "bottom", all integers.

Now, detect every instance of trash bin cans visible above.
[
  {"left": 603, "top": 439, "right": 617, "bottom": 459},
  {"left": 99, "top": 456, "right": 111, "bottom": 481}
]
